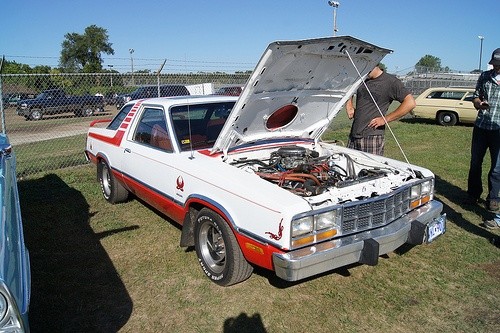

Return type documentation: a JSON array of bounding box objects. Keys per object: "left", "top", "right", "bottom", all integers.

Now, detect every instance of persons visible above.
[
  {"left": 456, "top": 47, "right": 500, "bottom": 214},
  {"left": 122, "top": 94, "right": 133, "bottom": 103},
  {"left": 112, "top": 92, "right": 119, "bottom": 98},
  {"left": 344, "top": 61, "right": 417, "bottom": 157},
  {"left": 483, "top": 213, "right": 500, "bottom": 230},
  {"left": 94, "top": 91, "right": 105, "bottom": 112}
]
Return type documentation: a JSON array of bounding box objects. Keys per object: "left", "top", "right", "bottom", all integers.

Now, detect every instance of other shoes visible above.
[
  {"left": 487, "top": 199, "right": 498, "bottom": 210},
  {"left": 468, "top": 197, "right": 480, "bottom": 206}
]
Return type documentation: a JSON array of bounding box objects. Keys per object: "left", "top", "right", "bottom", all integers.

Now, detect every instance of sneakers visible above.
[{"left": 484, "top": 219, "right": 500, "bottom": 230}]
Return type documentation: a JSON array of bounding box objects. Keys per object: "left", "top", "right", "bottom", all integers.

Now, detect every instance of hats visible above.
[{"left": 488, "top": 48, "right": 500, "bottom": 65}]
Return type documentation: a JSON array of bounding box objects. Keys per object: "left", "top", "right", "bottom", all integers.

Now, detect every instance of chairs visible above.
[{"left": 151, "top": 124, "right": 172, "bottom": 151}]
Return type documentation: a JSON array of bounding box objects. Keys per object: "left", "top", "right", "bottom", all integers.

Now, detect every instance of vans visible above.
[
  {"left": 116, "top": 85, "right": 190, "bottom": 109},
  {"left": 2, "top": 93, "right": 26, "bottom": 109}
]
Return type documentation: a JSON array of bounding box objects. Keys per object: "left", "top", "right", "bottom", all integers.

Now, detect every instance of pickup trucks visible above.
[{"left": 16, "top": 89, "right": 103, "bottom": 121}]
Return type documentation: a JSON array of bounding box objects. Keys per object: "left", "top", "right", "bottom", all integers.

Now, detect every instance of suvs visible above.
[{"left": 210, "top": 86, "right": 242, "bottom": 96}]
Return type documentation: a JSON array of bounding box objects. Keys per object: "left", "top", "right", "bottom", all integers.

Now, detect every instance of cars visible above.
[
  {"left": 82, "top": 34, "right": 448, "bottom": 286},
  {"left": 0, "top": 133, "right": 33, "bottom": 333},
  {"left": 410, "top": 86, "right": 479, "bottom": 126}
]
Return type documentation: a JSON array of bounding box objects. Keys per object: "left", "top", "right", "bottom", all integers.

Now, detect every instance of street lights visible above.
[
  {"left": 328, "top": 0, "right": 341, "bottom": 36},
  {"left": 108, "top": 65, "right": 114, "bottom": 87},
  {"left": 476, "top": 35, "right": 485, "bottom": 70},
  {"left": 129, "top": 48, "right": 135, "bottom": 79}
]
